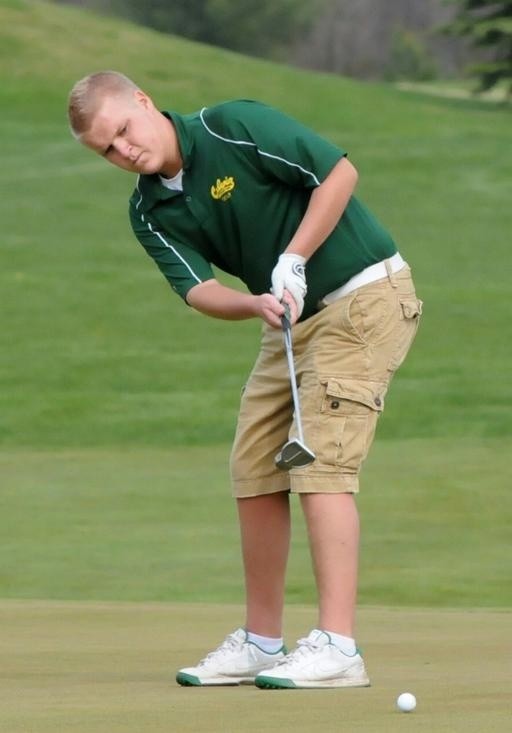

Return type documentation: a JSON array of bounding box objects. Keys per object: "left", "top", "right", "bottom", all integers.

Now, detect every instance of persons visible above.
[{"left": 68, "top": 70, "right": 424, "bottom": 688}]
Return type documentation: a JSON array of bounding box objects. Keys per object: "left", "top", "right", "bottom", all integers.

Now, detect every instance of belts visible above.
[{"left": 316, "top": 250, "right": 406, "bottom": 310}]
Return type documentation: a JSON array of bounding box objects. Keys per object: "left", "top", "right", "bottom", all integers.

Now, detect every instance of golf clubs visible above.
[{"left": 275, "top": 299, "right": 316, "bottom": 473}]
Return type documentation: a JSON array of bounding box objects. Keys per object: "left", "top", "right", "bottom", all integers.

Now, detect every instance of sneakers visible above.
[
  {"left": 255, "top": 629, "right": 369, "bottom": 688},
  {"left": 176, "top": 628, "right": 287, "bottom": 685}
]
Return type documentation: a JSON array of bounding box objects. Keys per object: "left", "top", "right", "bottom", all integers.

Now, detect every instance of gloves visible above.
[{"left": 270, "top": 253, "right": 308, "bottom": 319}]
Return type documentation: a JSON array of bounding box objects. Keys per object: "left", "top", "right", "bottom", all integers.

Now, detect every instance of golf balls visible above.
[{"left": 398, "top": 693, "right": 416, "bottom": 711}]
